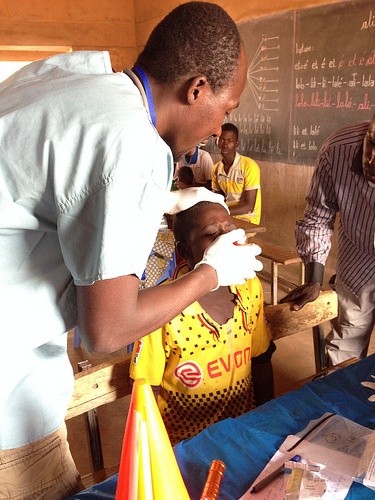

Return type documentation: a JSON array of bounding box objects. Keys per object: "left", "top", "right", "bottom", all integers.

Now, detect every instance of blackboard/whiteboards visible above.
[{"left": 198, "top": 0, "right": 375, "bottom": 167}]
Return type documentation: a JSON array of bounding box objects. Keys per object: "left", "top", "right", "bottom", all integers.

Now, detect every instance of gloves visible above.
[
  {"left": 164, "top": 186, "right": 230, "bottom": 217},
  {"left": 194, "top": 228, "right": 263, "bottom": 292}
]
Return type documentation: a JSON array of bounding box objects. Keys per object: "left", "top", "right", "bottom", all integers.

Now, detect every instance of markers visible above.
[{"left": 250, "top": 455, "right": 300, "bottom": 494}]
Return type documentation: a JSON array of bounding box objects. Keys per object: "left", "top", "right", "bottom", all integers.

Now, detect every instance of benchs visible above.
[
  {"left": 248, "top": 234, "right": 307, "bottom": 305},
  {"left": 61, "top": 288, "right": 340, "bottom": 490}
]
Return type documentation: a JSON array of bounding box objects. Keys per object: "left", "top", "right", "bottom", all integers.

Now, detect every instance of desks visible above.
[
  {"left": 58, "top": 354, "right": 375, "bottom": 500},
  {"left": 229, "top": 216, "right": 266, "bottom": 240}
]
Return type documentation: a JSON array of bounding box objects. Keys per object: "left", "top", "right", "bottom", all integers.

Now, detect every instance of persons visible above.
[
  {"left": 130, "top": 202, "right": 274, "bottom": 449},
  {"left": 277, "top": 117, "right": 375, "bottom": 374},
  {"left": 173, "top": 142, "right": 217, "bottom": 191},
  {"left": 1, "top": 1, "right": 265, "bottom": 500},
  {"left": 208, "top": 123, "right": 263, "bottom": 237}
]
